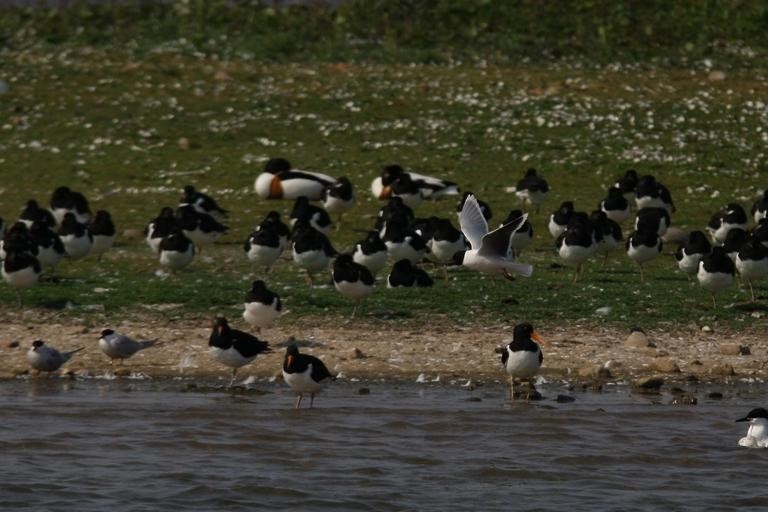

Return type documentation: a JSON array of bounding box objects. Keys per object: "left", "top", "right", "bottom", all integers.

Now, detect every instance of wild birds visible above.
[
  {"left": 243, "top": 156, "right": 535, "bottom": 324},
  {"left": 548, "top": 169, "right": 768, "bottom": 312},
  {"left": 281, "top": 345, "right": 341, "bottom": 409},
  {"left": 92, "top": 327, "right": 161, "bottom": 368},
  {"left": 0, "top": 181, "right": 119, "bottom": 308},
  {"left": 513, "top": 165, "right": 547, "bottom": 211},
  {"left": 138, "top": 181, "right": 231, "bottom": 275},
  {"left": 735, "top": 406, "right": 768, "bottom": 448},
  {"left": 491, "top": 320, "right": 548, "bottom": 403},
  {"left": 205, "top": 313, "right": 276, "bottom": 393},
  {"left": 28, "top": 338, "right": 90, "bottom": 382}
]
